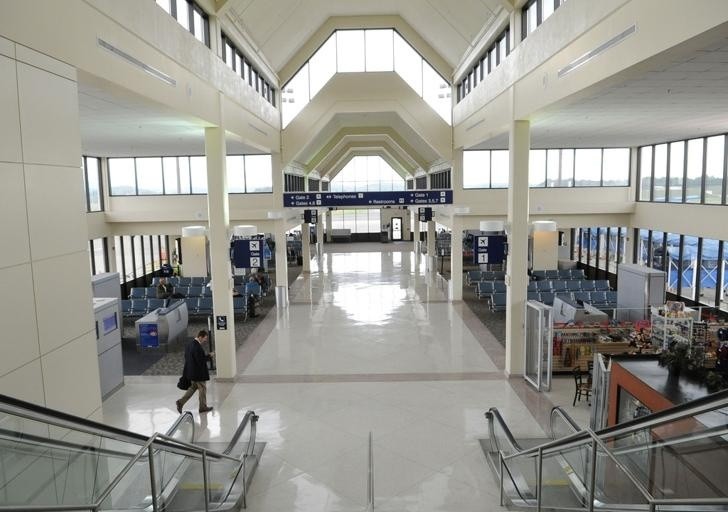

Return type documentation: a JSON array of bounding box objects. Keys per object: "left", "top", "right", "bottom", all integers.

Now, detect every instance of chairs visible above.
[
  {"left": 586, "top": 360, "right": 593, "bottom": 400},
  {"left": 466, "top": 270, "right": 617, "bottom": 312},
  {"left": 121, "top": 232, "right": 303, "bottom": 323},
  {"left": 572, "top": 366, "right": 593, "bottom": 406}
]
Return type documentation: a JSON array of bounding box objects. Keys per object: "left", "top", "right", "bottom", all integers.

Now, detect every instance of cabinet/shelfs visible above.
[{"left": 650, "top": 309, "right": 728, "bottom": 371}]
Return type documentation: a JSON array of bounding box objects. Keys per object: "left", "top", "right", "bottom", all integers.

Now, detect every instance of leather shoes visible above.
[
  {"left": 176, "top": 401, "right": 183, "bottom": 413},
  {"left": 199, "top": 407, "right": 213, "bottom": 413}
]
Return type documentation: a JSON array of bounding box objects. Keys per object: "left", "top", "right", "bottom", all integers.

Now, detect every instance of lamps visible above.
[
  {"left": 528, "top": 221, "right": 556, "bottom": 235},
  {"left": 267, "top": 212, "right": 282, "bottom": 220},
  {"left": 455, "top": 207, "right": 472, "bottom": 214},
  {"left": 479, "top": 220, "right": 511, "bottom": 235},
  {"left": 224, "top": 225, "right": 258, "bottom": 240},
  {"left": 182, "top": 226, "right": 210, "bottom": 240}
]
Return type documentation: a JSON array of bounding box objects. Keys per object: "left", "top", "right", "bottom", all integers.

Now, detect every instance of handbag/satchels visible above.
[{"left": 177, "top": 377, "right": 191, "bottom": 390}]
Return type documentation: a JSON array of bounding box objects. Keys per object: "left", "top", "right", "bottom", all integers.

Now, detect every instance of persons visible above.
[
  {"left": 245, "top": 278, "right": 260, "bottom": 318},
  {"left": 157, "top": 277, "right": 173, "bottom": 299},
  {"left": 175, "top": 330, "right": 216, "bottom": 414},
  {"left": 159, "top": 263, "right": 173, "bottom": 278},
  {"left": 248, "top": 266, "right": 266, "bottom": 290}
]
